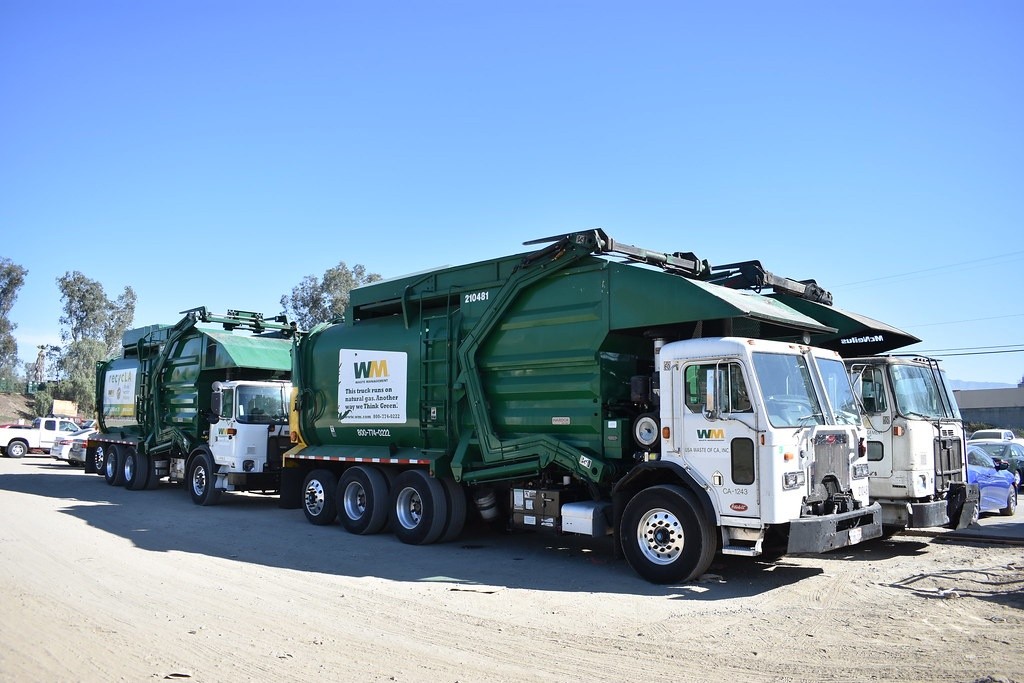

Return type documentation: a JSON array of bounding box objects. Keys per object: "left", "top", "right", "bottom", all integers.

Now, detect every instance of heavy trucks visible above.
[
  {"left": 84, "top": 305, "right": 298, "bottom": 507},
  {"left": 280, "top": 226, "right": 885, "bottom": 587},
  {"left": 712, "top": 259, "right": 981, "bottom": 542}
]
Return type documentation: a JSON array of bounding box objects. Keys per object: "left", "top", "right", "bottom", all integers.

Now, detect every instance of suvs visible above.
[{"left": 967, "top": 428, "right": 1016, "bottom": 444}]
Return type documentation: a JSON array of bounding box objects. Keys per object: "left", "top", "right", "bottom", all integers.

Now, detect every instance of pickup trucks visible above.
[{"left": 0, "top": 416, "right": 82, "bottom": 459}]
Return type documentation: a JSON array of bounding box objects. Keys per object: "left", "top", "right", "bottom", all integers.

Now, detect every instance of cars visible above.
[
  {"left": 0, "top": 423, "right": 44, "bottom": 455},
  {"left": 967, "top": 445, "right": 1018, "bottom": 525},
  {"left": 69, "top": 430, "right": 95, "bottom": 467},
  {"left": 50, "top": 427, "right": 98, "bottom": 467},
  {"left": 77, "top": 418, "right": 100, "bottom": 431},
  {"left": 973, "top": 441, "right": 1024, "bottom": 494}
]
